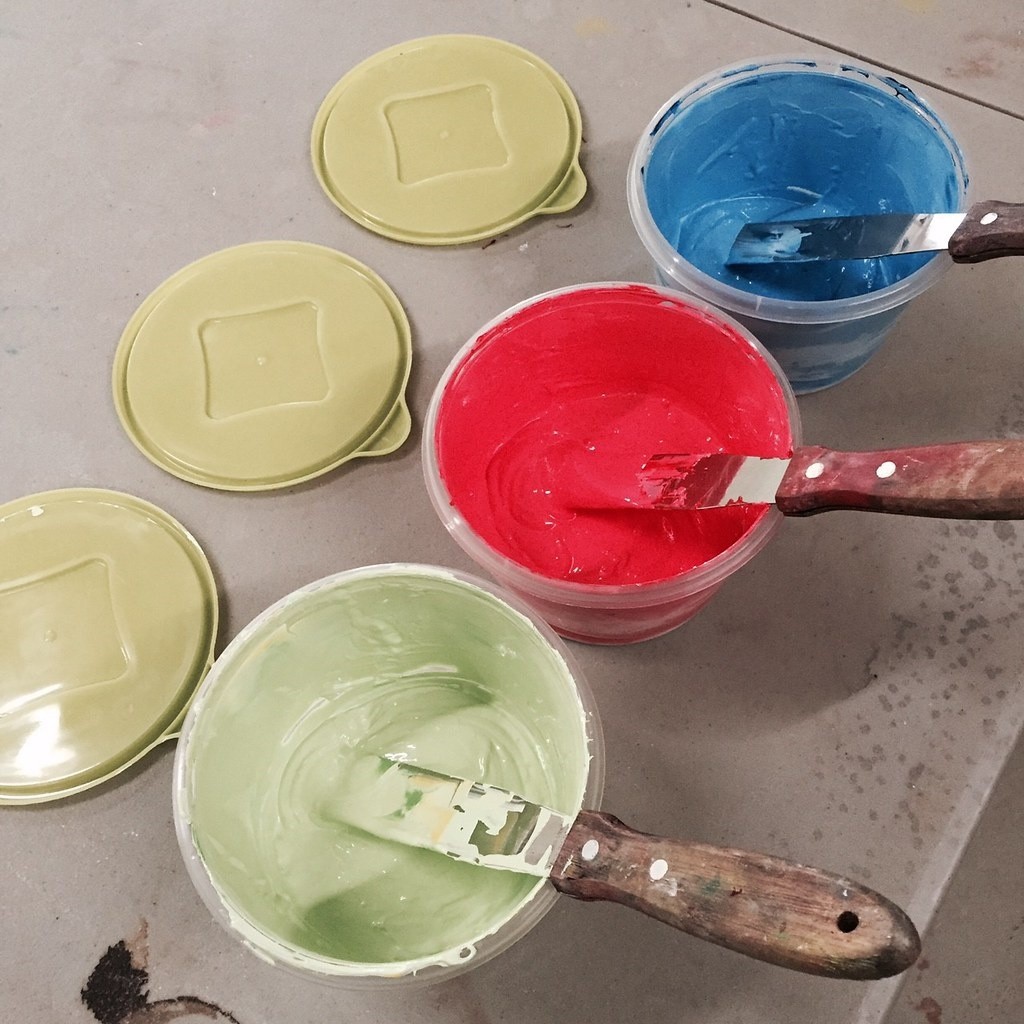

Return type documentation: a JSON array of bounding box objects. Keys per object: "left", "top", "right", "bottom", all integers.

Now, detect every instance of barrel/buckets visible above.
[
  {"left": 420, "top": 282, "right": 804, "bottom": 646},
  {"left": 625, "top": 51, "right": 970, "bottom": 395},
  {"left": 169, "top": 563, "right": 605, "bottom": 995}
]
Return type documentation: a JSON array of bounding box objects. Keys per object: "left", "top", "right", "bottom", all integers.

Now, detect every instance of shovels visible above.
[
  {"left": 724, "top": 200, "right": 1024, "bottom": 264},
  {"left": 322, "top": 753, "right": 923, "bottom": 982},
  {"left": 556, "top": 437, "right": 1023, "bottom": 519}
]
